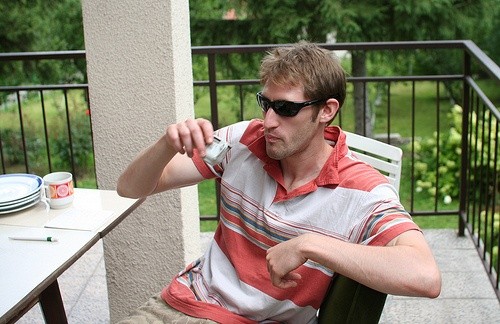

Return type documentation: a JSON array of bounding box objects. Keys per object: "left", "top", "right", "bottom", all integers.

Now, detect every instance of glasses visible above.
[{"left": 255, "top": 91, "right": 342, "bottom": 117}]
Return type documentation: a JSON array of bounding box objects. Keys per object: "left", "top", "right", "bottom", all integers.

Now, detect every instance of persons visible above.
[{"left": 111, "top": 42, "right": 443, "bottom": 324}]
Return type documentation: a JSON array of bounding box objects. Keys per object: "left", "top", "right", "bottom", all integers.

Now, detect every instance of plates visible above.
[{"left": 0, "top": 173, "right": 43, "bottom": 214}]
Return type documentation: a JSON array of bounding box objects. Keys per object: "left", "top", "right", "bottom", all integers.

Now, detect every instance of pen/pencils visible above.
[{"left": 8, "top": 236, "right": 58, "bottom": 241}]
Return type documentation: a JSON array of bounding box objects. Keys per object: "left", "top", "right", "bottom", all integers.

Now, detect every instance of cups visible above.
[
  {"left": 201, "top": 136, "right": 232, "bottom": 166},
  {"left": 42, "top": 172, "right": 74, "bottom": 209}
]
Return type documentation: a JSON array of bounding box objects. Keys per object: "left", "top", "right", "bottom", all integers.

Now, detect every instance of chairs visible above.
[{"left": 317, "top": 131, "right": 403, "bottom": 324}]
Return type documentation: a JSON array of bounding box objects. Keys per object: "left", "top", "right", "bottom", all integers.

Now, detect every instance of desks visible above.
[{"left": 0, "top": 170, "right": 146, "bottom": 324}]
